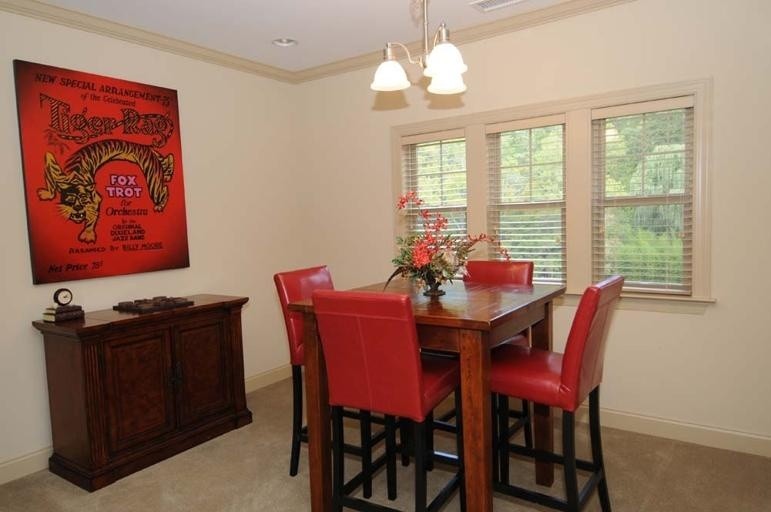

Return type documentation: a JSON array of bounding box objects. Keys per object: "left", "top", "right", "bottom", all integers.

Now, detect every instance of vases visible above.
[{"left": 383, "top": 191, "right": 512, "bottom": 289}]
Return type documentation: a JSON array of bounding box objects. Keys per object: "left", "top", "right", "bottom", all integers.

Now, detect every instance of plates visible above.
[{"left": 33, "top": 294, "right": 253, "bottom": 492}]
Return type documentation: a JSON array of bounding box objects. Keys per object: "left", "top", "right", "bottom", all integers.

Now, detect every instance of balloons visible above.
[{"left": 56, "top": 289, "right": 73, "bottom": 305}]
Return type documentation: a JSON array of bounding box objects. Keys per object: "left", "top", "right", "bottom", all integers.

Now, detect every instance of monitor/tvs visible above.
[{"left": 43, "top": 305, "right": 84, "bottom": 323}]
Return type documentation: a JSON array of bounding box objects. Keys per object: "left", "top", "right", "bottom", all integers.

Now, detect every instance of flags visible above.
[{"left": 424, "top": 284, "right": 446, "bottom": 296}]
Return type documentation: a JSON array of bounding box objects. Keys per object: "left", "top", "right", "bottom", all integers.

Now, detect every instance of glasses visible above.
[{"left": 371, "top": 0, "right": 468, "bottom": 94}]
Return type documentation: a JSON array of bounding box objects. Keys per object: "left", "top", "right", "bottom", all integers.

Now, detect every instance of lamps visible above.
[
  {"left": 475, "top": 264, "right": 477, "bottom": 264},
  {"left": 489, "top": 273, "right": 624, "bottom": 511},
  {"left": 463, "top": 261, "right": 534, "bottom": 286},
  {"left": 274, "top": 264, "right": 410, "bottom": 498},
  {"left": 312, "top": 289, "right": 467, "bottom": 511}
]
